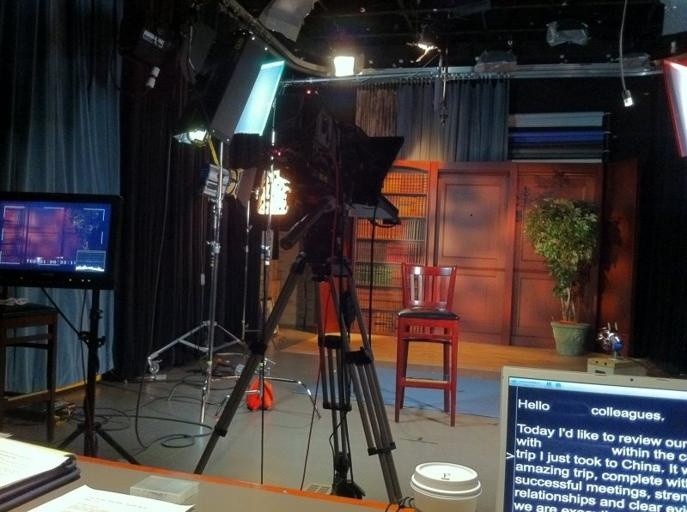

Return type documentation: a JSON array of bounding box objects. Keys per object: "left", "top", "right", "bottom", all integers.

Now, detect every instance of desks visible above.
[{"left": 8, "top": 445, "right": 418, "bottom": 511}]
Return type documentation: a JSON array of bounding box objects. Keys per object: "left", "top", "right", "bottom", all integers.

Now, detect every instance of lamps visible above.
[
  {"left": 416, "top": 0, "right": 441, "bottom": 51},
  {"left": 619, "top": 0, "right": 634, "bottom": 108},
  {"left": 333, "top": 53, "right": 355, "bottom": 77}
]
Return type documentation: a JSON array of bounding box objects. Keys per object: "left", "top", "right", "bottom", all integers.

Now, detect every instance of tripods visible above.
[
  {"left": 56, "top": 293, "right": 140, "bottom": 467},
  {"left": 193, "top": 254, "right": 402, "bottom": 505}
]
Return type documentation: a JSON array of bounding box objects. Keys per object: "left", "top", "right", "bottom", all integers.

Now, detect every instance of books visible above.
[{"left": 0, "top": 436, "right": 82, "bottom": 511}]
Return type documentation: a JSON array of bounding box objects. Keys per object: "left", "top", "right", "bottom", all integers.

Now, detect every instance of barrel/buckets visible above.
[{"left": 408, "top": 462, "right": 484, "bottom": 511}]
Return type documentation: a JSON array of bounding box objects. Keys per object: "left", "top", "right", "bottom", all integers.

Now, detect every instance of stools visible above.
[{"left": 0, "top": 298, "right": 59, "bottom": 442}]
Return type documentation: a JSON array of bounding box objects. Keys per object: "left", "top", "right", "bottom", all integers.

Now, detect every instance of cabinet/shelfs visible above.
[{"left": 347, "top": 159, "right": 431, "bottom": 335}]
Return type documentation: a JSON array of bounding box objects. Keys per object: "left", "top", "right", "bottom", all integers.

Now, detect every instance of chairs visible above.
[{"left": 395, "top": 261, "right": 460, "bottom": 427}]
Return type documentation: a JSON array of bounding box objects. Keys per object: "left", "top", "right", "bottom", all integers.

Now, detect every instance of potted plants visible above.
[{"left": 521, "top": 197, "right": 604, "bottom": 356}]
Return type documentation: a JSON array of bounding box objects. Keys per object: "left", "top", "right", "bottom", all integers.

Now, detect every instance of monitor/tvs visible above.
[{"left": 0, "top": 188, "right": 123, "bottom": 290}]
